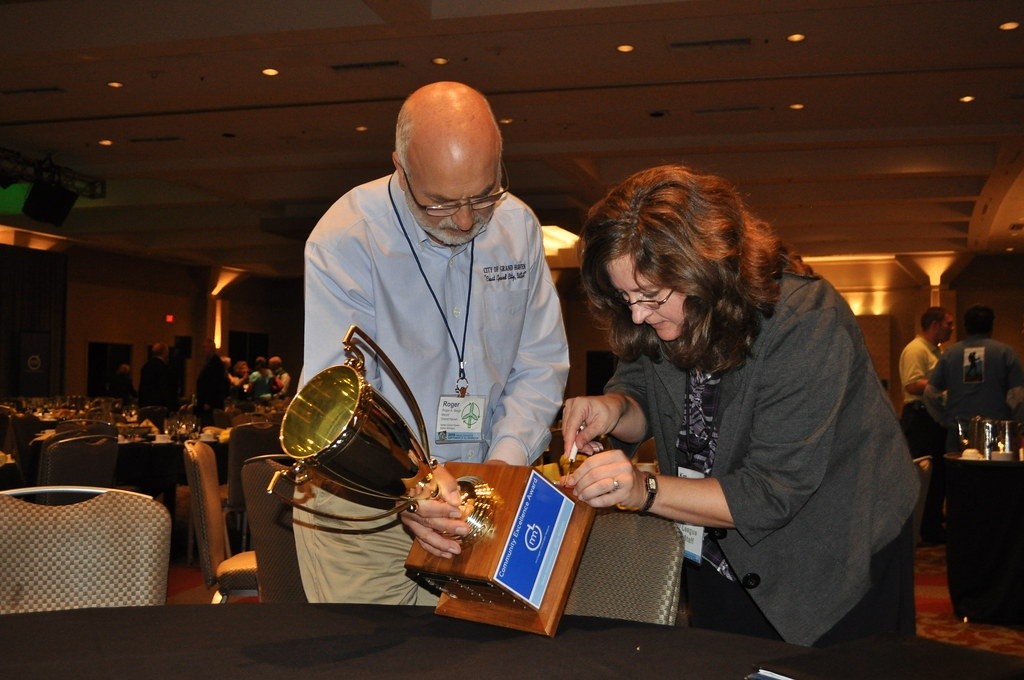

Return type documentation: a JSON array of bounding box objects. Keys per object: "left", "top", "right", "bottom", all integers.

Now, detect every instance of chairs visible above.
[{"left": 0, "top": 395, "right": 683, "bottom": 622}]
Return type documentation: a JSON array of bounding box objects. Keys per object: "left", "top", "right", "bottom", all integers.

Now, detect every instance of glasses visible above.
[
  {"left": 400, "top": 160, "right": 509, "bottom": 217},
  {"left": 611, "top": 287, "right": 676, "bottom": 313}
]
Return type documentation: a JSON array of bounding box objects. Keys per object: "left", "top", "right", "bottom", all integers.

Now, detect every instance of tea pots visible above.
[{"left": 953, "top": 414, "right": 1021, "bottom": 458}]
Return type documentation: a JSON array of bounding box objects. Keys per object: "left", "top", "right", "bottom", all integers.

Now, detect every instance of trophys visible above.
[{"left": 266, "top": 324, "right": 596, "bottom": 638}]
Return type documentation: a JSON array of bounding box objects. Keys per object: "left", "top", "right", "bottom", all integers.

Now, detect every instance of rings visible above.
[{"left": 612, "top": 481, "right": 619, "bottom": 489}]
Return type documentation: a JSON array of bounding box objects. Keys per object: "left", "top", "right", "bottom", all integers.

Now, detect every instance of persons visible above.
[
  {"left": 111, "top": 341, "right": 290, "bottom": 427},
  {"left": 899, "top": 308, "right": 954, "bottom": 548},
  {"left": 289, "top": 83, "right": 571, "bottom": 604},
  {"left": 555, "top": 169, "right": 922, "bottom": 644},
  {"left": 923, "top": 305, "right": 1024, "bottom": 455}
]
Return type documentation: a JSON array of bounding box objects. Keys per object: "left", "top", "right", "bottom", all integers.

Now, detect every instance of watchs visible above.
[{"left": 637, "top": 470, "right": 657, "bottom": 513}]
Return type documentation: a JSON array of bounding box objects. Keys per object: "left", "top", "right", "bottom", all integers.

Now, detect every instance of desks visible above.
[
  {"left": 944, "top": 452, "right": 1024, "bottom": 632},
  {"left": 0, "top": 604, "right": 1024, "bottom": 680},
  {"left": 119, "top": 434, "right": 232, "bottom": 564}
]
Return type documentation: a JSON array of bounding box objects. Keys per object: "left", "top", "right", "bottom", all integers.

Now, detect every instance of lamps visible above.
[{"left": 534, "top": 206, "right": 581, "bottom": 257}]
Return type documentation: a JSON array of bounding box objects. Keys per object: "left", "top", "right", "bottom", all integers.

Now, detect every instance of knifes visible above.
[{"left": 563, "top": 424, "right": 589, "bottom": 486}]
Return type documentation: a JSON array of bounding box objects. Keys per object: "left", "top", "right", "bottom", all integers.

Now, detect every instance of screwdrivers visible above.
[{"left": 562, "top": 420, "right": 585, "bottom": 488}]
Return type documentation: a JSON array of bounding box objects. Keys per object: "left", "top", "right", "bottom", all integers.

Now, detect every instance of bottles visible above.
[{"left": 166, "top": 410, "right": 202, "bottom": 445}]
